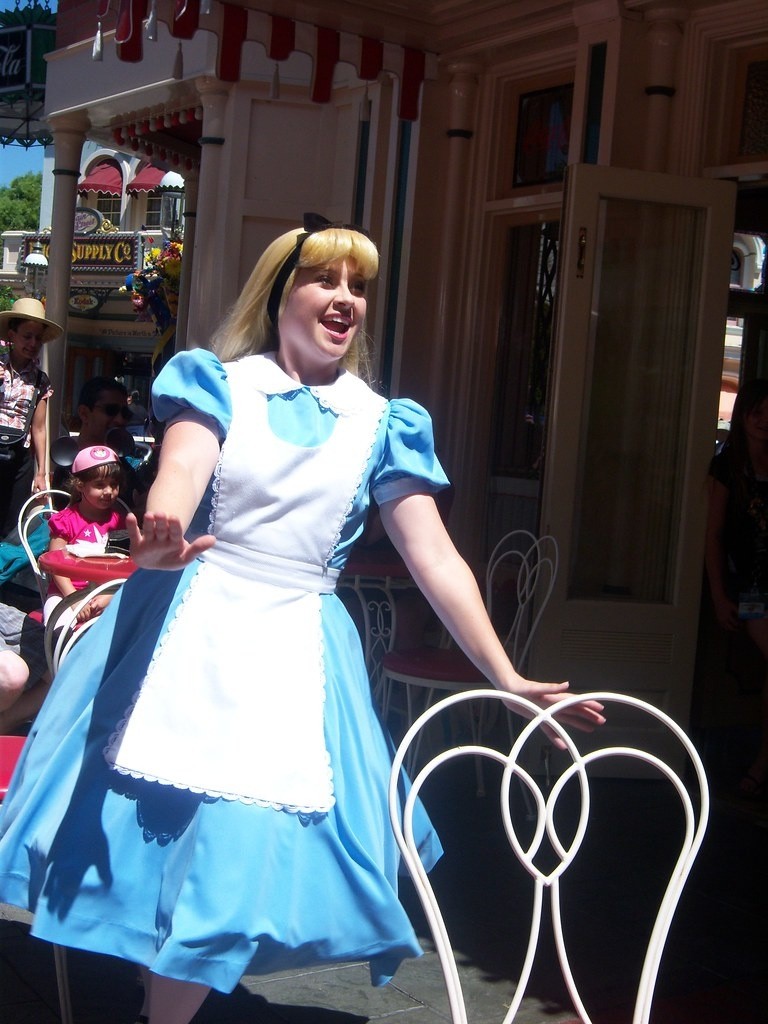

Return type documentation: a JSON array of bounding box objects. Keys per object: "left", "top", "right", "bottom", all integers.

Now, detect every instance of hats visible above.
[{"left": 0, "top": 298, "right": 64, "bottom": 344}]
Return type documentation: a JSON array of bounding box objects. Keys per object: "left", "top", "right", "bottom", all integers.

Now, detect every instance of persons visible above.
[
  {"left": 46, "top": 445, "right": 128, "bottom": 633},
  {"left": 125, "top": 388, "right": 147, "bottom": 427},
  {"left": 0, "top": 602, "right": 66, "bottom": 736},
  {"left": 694, "top": 379, "right": 768, "bottom": 797},
  {"left": 0, "top": 298, "right": 65, "bottom": 539},
  {"left": 52, "top": 374, "right": 146, "bottom": 510},
  {"left": 0, "top": 212, "right": 607, "bottom": 1024}
]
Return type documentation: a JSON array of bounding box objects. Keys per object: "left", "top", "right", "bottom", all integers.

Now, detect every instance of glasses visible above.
[{"left": 94, "top": 404, "right": 134, "bottom": 419}]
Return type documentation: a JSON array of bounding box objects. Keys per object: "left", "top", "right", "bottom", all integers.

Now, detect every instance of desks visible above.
[{"left": 36, "top": 545, "right": 411, "bottom": 580}]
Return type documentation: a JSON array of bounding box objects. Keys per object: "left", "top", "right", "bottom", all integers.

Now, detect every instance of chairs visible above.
[
  {"left": 17, "top": 490, "right": 562, "bottom": 822},
  {"left": 1, "top": 735, "right": 75, "bottom": 1024},
  {"left": 389, "top": 688, "right": 711, "bottom": 1024}
]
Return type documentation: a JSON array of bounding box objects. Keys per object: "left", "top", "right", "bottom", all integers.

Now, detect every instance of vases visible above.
[{"left": 142, "top": 282, "right": 178, "bottom": 335}]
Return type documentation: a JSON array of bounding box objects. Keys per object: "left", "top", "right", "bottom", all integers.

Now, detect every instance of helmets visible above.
[{"left": 72, "top": 446, "right": 122, "bottom": 475}]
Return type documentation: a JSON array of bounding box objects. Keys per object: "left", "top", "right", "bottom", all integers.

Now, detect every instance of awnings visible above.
[
  {"left": 78, "top": 159, "right": 123, "bottom": 200},
  {"left": 125, "top": 163, "right": 168, "bottom": 199}
]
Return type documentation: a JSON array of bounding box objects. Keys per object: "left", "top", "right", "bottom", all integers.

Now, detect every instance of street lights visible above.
[{"left": 155, "top": 172, "right": 187, "bottom": 236}]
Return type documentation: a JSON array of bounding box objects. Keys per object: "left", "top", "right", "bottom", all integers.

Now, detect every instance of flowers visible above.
[{"left": 118, "top": 225, "right": 184, "bottom": 312}]
